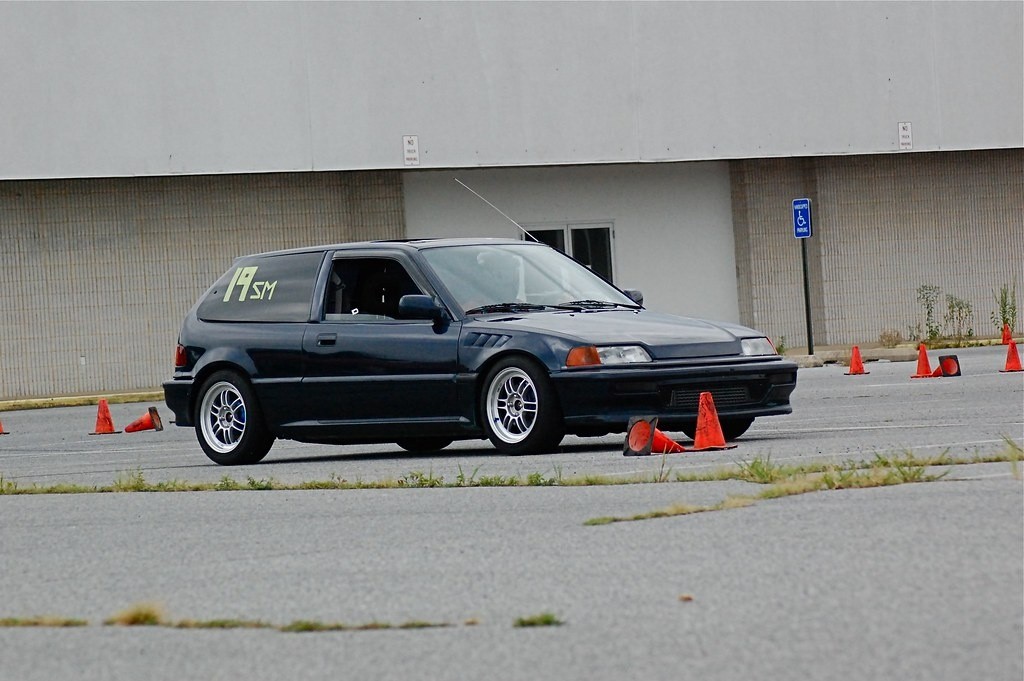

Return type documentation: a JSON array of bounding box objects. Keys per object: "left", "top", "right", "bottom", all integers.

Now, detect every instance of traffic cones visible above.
[
  {"left": 687, "top": 391, "right": 738, "bottom": 453},
  {"left": 623, "top": 416, "right": 687, "bottom": 457},
  {"left": 999, "top": 340, "right": 1024, "bottom": 372},
  {"left": 844, "top": 345, "right": 871, "bottom": 375},
  {"left": 931, "top": 355, "right": 961, "bottom": 377},
  {"left": 88, "top": 399, "right": 124, "bottom": 436},
  {"left": 998, "top": 323, "right": 1017, "bottom": 345},
  {"left": 909, "top": 344, "right": 934, "bottom": 379},
  {"left": 124, "top": 407, "right": 163, "bottom": 433}
]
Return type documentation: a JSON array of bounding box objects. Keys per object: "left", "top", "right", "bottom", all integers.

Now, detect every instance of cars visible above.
[{"left": 159, "top": 234, "right": 800, "bottom": 467}]
